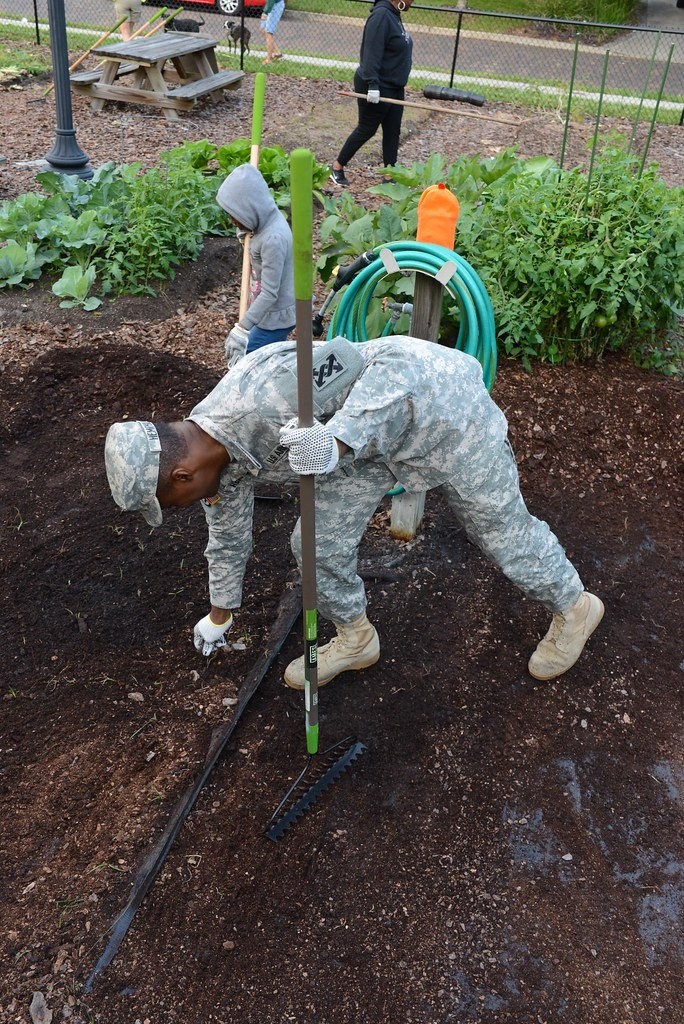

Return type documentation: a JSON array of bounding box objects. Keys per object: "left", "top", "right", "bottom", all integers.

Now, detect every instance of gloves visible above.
[
  {"left": 279, "top": 416, "right": 340, "bottom": 475},
  {"left": 237, "top": 230, "right": 254, "bottom": 244},
  {"left": 224, "top": 322, "right": 250, "bottom": 369},
  {"left": 367, "top": 90, "right": 380, "bottom": 104},
  {"left": 194, "top": 612, "right": 233, "bottom": 656}
]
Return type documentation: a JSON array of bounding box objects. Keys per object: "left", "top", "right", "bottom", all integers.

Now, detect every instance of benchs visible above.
[{"left": 69, "top": 63, "right": 246, "bottom": 124}]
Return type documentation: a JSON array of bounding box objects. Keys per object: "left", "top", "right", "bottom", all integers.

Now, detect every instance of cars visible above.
[{"left": 141, "top": 0, "right": 288, "bottom": 17}]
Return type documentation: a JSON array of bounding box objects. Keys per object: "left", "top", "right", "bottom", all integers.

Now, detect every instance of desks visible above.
[{"left": 87, "top": 31, "right": 225, "bottom": 123}]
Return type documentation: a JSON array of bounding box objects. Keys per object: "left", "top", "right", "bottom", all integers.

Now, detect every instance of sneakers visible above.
[
  {"left": 327, "top": 164, "right": 349, "bottom": 186},
  {"left": 381, "top": 178, "right": 396, "bottom": 184}
]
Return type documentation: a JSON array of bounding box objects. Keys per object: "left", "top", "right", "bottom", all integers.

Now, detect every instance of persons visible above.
[
  {"left": 105, "top": 334, "right": 604, "bottom": 690},
  {"left": 111, "top": 0, "right": 141, "bottom": 43},
  {"left": 215, "top": 163, "right": 316, "bottom": 360},
  {"left": 329, "top": 0, "right": 414, "bottom": 186},
  {"left": 258, "top": 0, "right": 285, "bottom": 65}
]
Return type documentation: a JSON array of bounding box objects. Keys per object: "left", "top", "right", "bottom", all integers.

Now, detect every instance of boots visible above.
[
  {"left": 283, "top": 608, "right": 380, "bottom": 690},
  {"left": 528, "top": 590, "right": 604, "bottom": 680}
]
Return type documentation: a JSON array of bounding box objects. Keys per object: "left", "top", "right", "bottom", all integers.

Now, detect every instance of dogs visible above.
[
  {"left": 161, "top": 12, "right": 205, "bottom": 33},
  {"left": 222, "top": 20, "right": 251, "bottom": 55}
]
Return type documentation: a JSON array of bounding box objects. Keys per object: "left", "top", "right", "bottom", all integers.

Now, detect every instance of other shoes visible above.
[{"left": 273, "top": 53, "right": 282, "bottom": 58}]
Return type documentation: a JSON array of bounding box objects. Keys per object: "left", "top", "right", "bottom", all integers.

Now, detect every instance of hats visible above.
[{"left": 105, "top": 420, "right": 163, "bottom": 528}]
